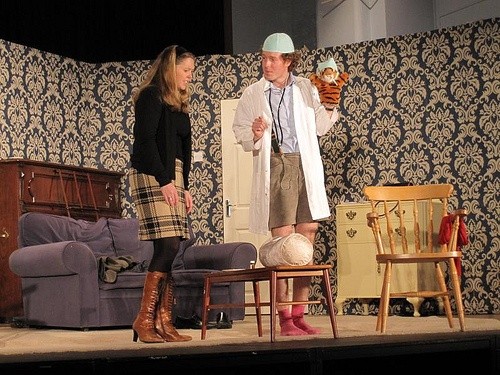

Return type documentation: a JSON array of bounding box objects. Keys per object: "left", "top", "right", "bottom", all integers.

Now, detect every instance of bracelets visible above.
[{"left": 326, "top": 108, "right": 334, "bottom": 110}]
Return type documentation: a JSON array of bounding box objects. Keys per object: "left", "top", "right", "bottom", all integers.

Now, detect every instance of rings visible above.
[{"left": 169, "top": 197, "right": 171, "bottom": 199}]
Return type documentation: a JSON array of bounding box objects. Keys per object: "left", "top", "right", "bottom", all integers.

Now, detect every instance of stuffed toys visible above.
[{"left": 310, "top": 58, "right": 350, "bottom": 106}]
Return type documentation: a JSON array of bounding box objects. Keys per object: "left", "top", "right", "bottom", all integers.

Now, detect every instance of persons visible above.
[
  {"left": 233, "top": 33, "right": 339, "bottom": 336},
  {"left": 129, "top": 44, "right": 194, "bottom": 346}
]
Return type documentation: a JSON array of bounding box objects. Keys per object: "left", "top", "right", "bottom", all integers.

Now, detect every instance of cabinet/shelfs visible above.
[
  {"left": 336, "top": 198, "right": 445, "bottom": 317},
  {"left": 0, "top": 158, "right": 126, "bottom": 324},
  {"left": 9, "top": 212, "right": 257, "bottom": 331}
]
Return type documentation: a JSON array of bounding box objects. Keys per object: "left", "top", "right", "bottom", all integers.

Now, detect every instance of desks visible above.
[{"left": 201, "top": 264, "right": 340, "bottom": 342}]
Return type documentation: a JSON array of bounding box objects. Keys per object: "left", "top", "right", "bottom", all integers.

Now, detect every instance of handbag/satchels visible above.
[{"left": 258, "top": 232, "right": 314, "bottom": 268}]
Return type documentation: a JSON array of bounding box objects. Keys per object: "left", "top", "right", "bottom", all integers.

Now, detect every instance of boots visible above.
[{"left": 131, "top": 269, "right": 192, "bottom": 343}]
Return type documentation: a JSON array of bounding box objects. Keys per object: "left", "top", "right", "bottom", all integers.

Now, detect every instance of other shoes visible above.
[
  {"left": 175, "top": 312, "right": 216, "bottom": 329},
  {"left": 216, "top": 311, "right": 232, "bottom": 329}
]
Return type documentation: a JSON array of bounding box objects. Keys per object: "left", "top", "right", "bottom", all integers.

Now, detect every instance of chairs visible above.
[{"left": 364, "top": 182, "right": 467, "bottom": 332}]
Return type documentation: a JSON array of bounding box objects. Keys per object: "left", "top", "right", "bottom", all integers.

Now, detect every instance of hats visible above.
[{"left": 262, "top": 32, "right": 295, "bottom": 53}]
